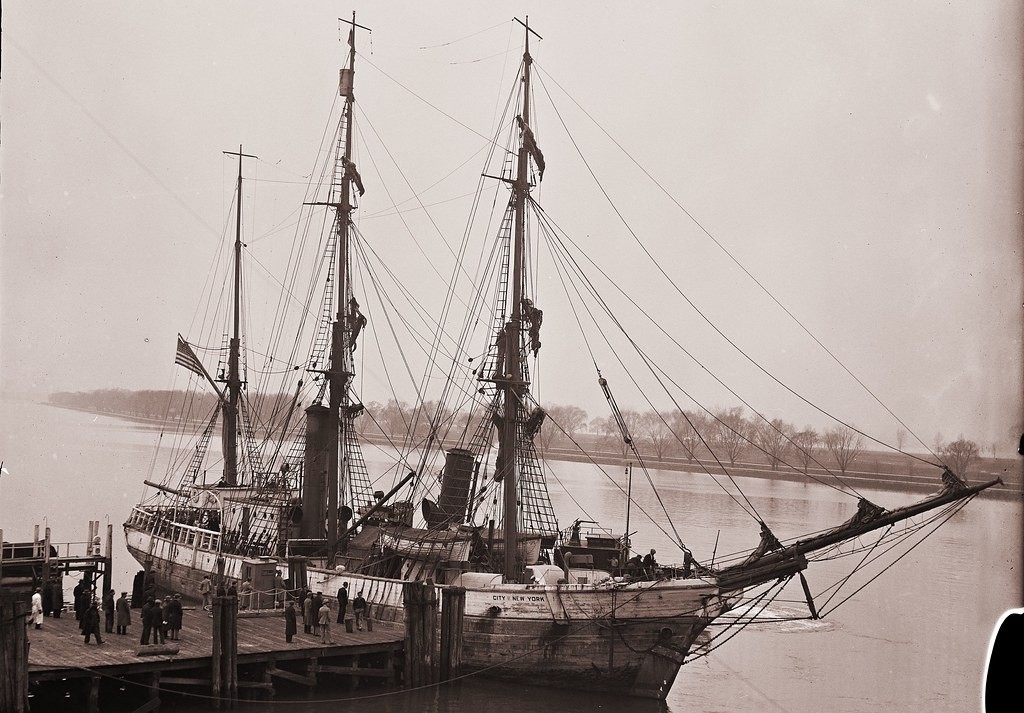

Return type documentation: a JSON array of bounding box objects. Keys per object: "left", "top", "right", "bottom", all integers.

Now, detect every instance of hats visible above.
[
  {"left": 82, "top": 590, "right": 91, "bottom": 595},
  {"left": 155, "top": 598, "right": 163, "bottom": 603},
  {"left": 163, "top": 595, "right": 173, "bottom": 601},
  {"left": 343, "top": 582, "right": 348, "bottom": 585},
  {"left": 78, "top": 579, "right": 85, "bottom": 584},
  {"left": 315, "top": 592, "right": 323, "bottom": 595},
  {"left": 91, "top": 602, "right": 100, "bottom": 607},
  {"left": 39, "top": 577, "right": 62, "bottom": 583},
  {"left": 34, "top": 587, "right": 42, "bottom": 592},
  {"left": 173, "top": 593, "right": 182, "bottom": 599},
  {"left": 321, "top": 599, "right": 329, "bottom": 605}
]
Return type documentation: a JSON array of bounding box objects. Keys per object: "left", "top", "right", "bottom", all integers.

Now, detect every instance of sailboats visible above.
[{"left": 122, "top": 7, "right": 1006, "bottom": 700}]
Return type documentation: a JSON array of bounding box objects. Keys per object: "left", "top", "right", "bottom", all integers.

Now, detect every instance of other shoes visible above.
[
  {"left": 84, "top": 641, "right": 89, "bottom": 644},
  {"left": 171, "top": 638, "right": 181, "bottom": 641},
  {"left": 98, "top": 640, "right": 106, "bottom": 645}
]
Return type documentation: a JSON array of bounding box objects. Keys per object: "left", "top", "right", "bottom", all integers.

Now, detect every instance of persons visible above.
[
  {"left": 607, "top": 555, "right": 619, "bottom": 576},
  {"left": 643, "top": 549, "right": 656, "bottom": 573},
  {"left": 623, "top": 555, "right": 642, "bottom": 575},
  {"left": 227, "top": 581, "right": 238, "bottom": 612},
  {"left": 140, "top": 594, "right": 182, "bottom": 644},
  {"left": 299, "top": 585, "right": 336, "bottom": 645},
  {"left": 337, "top": 582, "right": 348, "bottom": 624},
  {"left": 240, "top": 577, "right": 253, "bottom": 610},
  {"left": 74, "top": 579, "right": 90, "bottom": 630},
  {"left": 116, "top": 592, "right": 131, "bottom": 635},
  {"left": 82, "top": 601, "right": 105, "bottom": 645},
  {"left": 200, "top": 575, "right": 211, "bottom": 609},
  {"left": 217, "top": 582, "right": 226, "bottom": 597},
  {"left": 102, "top": 589, "right": 115, "bottom": 633},
  {"left": 285, "top": 601, "right": 297, "bottom": 642},
  {"left": 275, "top": 573, "right": 286, "bottom": 607},
  {"left": 353, "top": 592, "right": 365, "bottom": 631},
  {"left": 31, "top": 587, "right": 44, "bottom": 629}
]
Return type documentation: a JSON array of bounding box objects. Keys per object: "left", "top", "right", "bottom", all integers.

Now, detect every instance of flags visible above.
[{"left": 174, "top": 338, "right": 204, "bottom": 377}]
